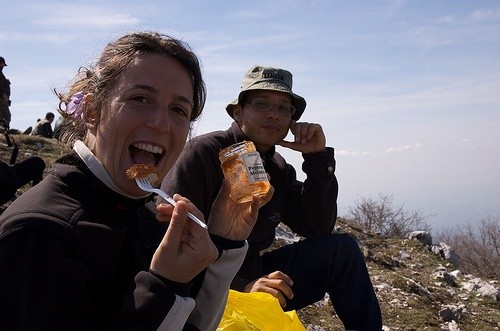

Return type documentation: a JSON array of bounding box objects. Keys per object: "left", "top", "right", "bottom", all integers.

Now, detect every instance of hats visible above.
[{"left": 225, "top": 65, "right": 307, "bottom": 123}]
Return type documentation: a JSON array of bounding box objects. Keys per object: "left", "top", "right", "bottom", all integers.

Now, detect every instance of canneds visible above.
[{"left": 219, "top": 140, "right": 270, "bottom": 204}]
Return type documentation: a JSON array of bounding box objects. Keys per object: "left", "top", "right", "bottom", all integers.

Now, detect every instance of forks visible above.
[{"left": 135, "top": 177, "right": 208, "bottom": 229}]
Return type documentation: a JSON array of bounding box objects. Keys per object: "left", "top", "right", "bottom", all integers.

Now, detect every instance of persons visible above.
[
  {"left": 0, "top": 30, "right": 274, "bottom": 331},
  {"left": 0, "top": 156, "right": 46, "bottom": 206},
  {"left": 32, "top": 112, "right": 54, "bottom": 138},
  {"left": 157, "top": 65, "right": 384, "bottom": 331},
  {"left": 0, "top": 56, "right": 12, "bottom": 133},
  {"left": 54, "top": 115, "right": 69, "bottom": 139}
]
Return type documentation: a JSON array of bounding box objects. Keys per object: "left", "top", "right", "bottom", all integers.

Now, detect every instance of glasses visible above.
[{"left": 244, "top": 98, "right": 295, "bottom": 117}]
